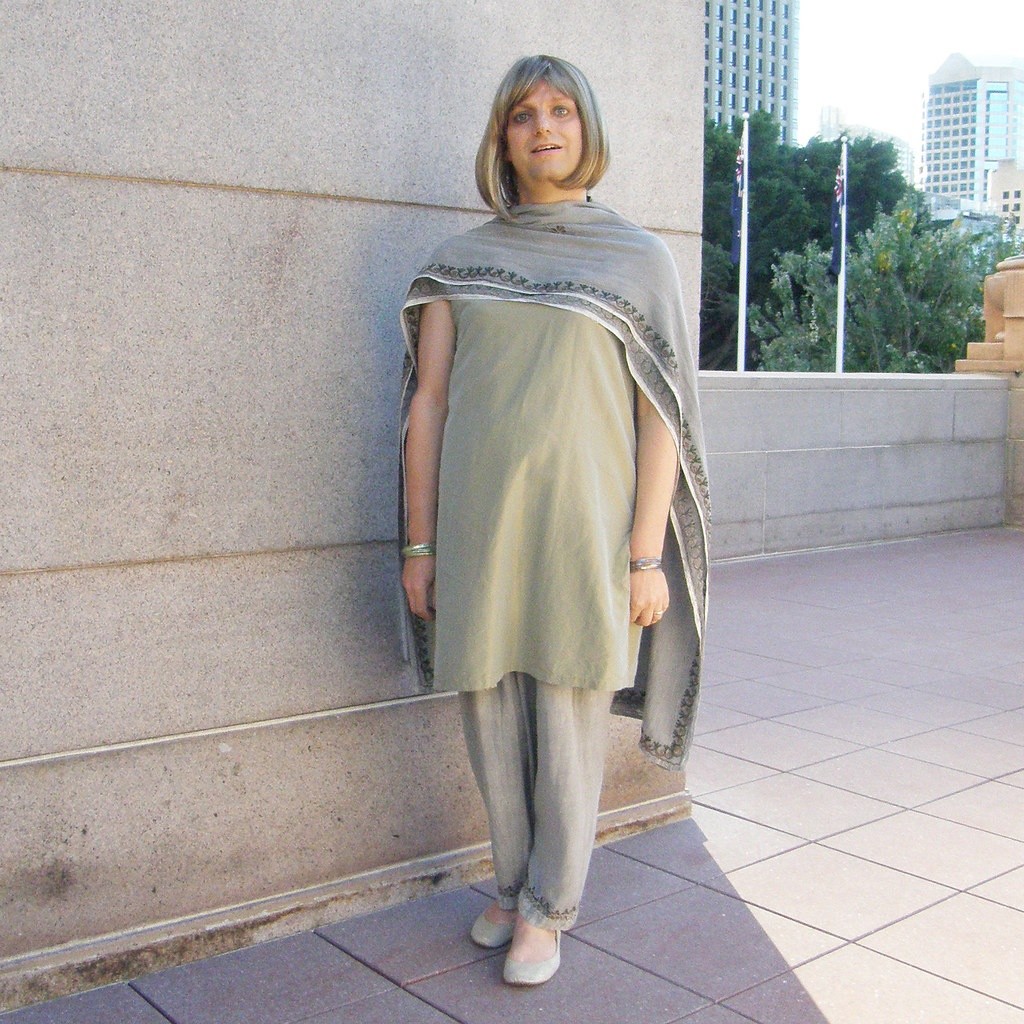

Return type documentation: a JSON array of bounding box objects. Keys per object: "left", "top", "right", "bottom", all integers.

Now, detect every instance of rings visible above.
[{"left": 653, "top": 610, "right": 664, "bottom": 615}]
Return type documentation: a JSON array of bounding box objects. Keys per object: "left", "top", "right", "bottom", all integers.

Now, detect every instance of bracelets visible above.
[
  {"left": 400, "top": 542, "right": 436, "bottom": 558},
  {"left": 629, "top": 557, "right": 662, "bottom": 573}
]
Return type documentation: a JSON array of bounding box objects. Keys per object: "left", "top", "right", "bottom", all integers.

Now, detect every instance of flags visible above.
[
  {"left": 825, "top": 153, "right": 844, "bottom": 287},
  {"left": 731, "top": 126, "right": 745, "bottom": 271}
]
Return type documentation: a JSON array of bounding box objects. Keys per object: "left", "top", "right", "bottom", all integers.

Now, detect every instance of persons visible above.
[{"left": 394, "top": 53, "right": 714, "bottom": 988}]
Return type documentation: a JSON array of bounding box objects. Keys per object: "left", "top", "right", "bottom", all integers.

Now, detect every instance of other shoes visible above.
[
  {"left": 470, "top": 899, "right": 517, "bottom": 948},
  {"left": 504, "top": 928, "right": 562, "bottom": 987}
]
